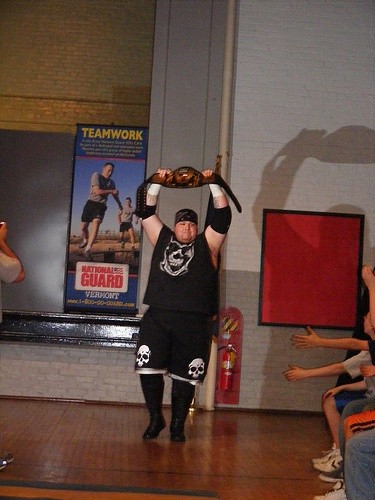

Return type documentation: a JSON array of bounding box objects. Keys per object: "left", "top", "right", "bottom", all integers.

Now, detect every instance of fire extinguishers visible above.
[{"left": 218, "top": 343, "right": 238, "bottom": 392}]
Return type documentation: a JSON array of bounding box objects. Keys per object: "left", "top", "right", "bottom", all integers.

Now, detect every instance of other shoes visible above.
[
  {"left": 79, "top": 242, "right": 87, "bottom": 248},
  {"left": 130, "top": 245, "right": 135, "bottom": 250},
  {"left": 121, "top": 241, "right": 125, "bottom": 248},
  {"left": 313, "top": 480, "right": 346, "bottom": 500},
  {"left": 318, "top": 465, "right": 344, "bottom": 483},
  {"left": 311, "top": 443, "right": 343, "bottom": 471},
  {"left": 0, "top": 452, "right": 14, "bottom": 470},
  {"left": 85, "top": 249, "right": 90, "bottom": 255}
]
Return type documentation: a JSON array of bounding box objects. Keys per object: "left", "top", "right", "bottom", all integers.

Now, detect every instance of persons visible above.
[
  {"left": 79, "top": 163, "right": 123, "bottom": 258},
  {"left": 134, "top": 167, "right": 232, "bottom": 442},
  {"left": 118, "top": 197, "right": 138, "bottom": 250},
  {"left": 0, "top": 221, "right": 27, "bottom": 470},
  {"left": 282, "top": 265, "right": 375, "bottom": 500}
]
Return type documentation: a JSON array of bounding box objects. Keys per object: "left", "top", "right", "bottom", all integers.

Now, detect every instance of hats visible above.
[{"left": 174, "top": 208, "right": 198, "bottom": 225}]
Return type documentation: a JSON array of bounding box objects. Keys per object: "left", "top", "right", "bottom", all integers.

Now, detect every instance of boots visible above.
[
  {"left": 137, "top": 379, "right": 166, "bottom": 440},
  {"left": 170, "top": 381, "right": 194, "bottom": 442}
]
